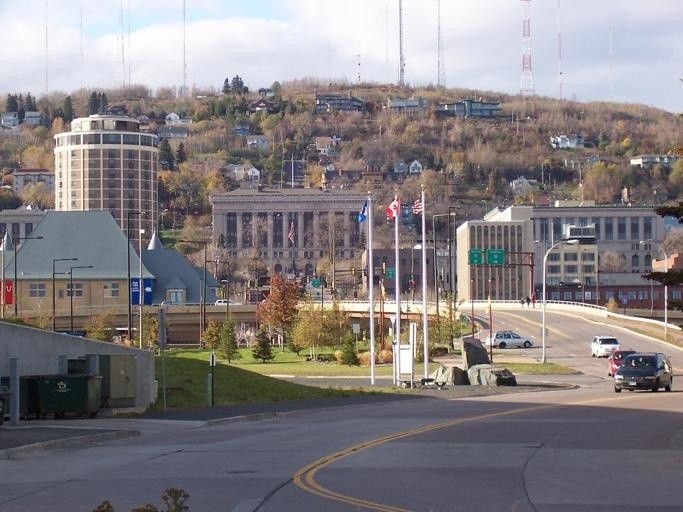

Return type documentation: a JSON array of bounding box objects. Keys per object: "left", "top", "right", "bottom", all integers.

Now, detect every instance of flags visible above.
[
  {"left": 359, "top": 199, "right": 368, "bottom": 224},
  {"left": 287, "top": 221, "right": 295, "bottom": 245},
  {"left": 383, "top": 194, "right": 401, "bottom": 222},
  {"left": 412, "top": 192, "right": 422, "bottom": 215}
]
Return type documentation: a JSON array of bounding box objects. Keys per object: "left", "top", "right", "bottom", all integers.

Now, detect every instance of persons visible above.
[
  {"left": 529, "top": 295, "right": 536, "bottom": 309},
  {"left": 626, "top": 358, "right": 638, "bottom": 367},
  {"left": 525, "top": 295, "right": 530, "bottom": 308},
  {"left": 520, "top": 298, "right": 524, "bottom": 308}
]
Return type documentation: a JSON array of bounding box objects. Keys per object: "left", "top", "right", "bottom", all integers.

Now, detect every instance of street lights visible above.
[
  {"left": 541, "top": 239, "right": 579, "bottom": 364},
  {"left": 53, "top": 258, "right": 93, "bottom": 331},
  {"left": 433, "top": 206, "right": 460, "bottom": 349},
  {"left": 178, "top": 240, "right": 229, "bottom": 348},
  {"left": 127, "top": 211, "right": 148, "bottom": 340},
  {"left": 639, "top": 241, "right": 667, "bottom": 336},
  {"left": 14, "top": 236, "right": 43, "bottom": 317}
]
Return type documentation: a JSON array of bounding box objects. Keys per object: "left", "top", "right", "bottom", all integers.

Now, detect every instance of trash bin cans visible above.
[{"left": 0, "top": 374, "right": 104, "bottom": 421}]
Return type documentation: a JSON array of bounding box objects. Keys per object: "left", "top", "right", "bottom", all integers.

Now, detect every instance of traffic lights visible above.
[{"left": 354, "top": 292, "right": 357, "bottom": 298}]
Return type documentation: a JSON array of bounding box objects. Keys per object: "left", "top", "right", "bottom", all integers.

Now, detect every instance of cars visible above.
[
  {"left": 591, "top": 335, "right": 672, "bottom": 392},
  {"left": 161, "top": 301, "right": 178, "bottom": 306},
  {"left": 215, "top": 300, "right": 242, "bottom": 305},
  {"left": 486, "top": 331, "right": 533, "bottom": 349}
]
